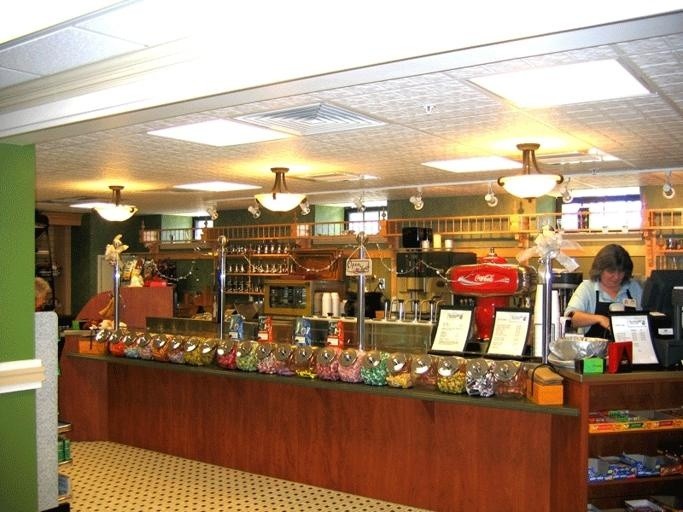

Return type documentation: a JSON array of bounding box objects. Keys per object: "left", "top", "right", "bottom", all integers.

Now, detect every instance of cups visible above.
[
  {"left": 601, "top": 226, "right": 607, "bottom": 233},
  {"left": 444, "top": 239, "right": 453, "bottom": 252},
  {"left": 622, "top": 226, "right": 628, "bottom": 234},
  {"left": 313, "top": 291, "right": 347, "bottom": 318},
  {"left": 432, "top": 234, "right": 441, "bottom": 250},
  {"left": 654, "top": 231, "right": 683, "bottom": 271}
]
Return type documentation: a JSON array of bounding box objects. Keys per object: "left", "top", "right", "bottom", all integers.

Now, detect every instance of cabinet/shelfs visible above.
[
  {"left": 550, "top": 368, "right": 683, "bottom": 512},
  {"left": 224, "top": 254, "right": 289, "bottom": 292}
]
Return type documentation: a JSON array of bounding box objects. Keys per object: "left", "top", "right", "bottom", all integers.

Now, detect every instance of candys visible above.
[{"left": 98, "top": 339, "right": 525, "bottom": 398}]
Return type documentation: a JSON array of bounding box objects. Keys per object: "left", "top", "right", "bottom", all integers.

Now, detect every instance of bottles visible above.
[
  {"left": 224, "top": 240, "right": 306, "bottom": 308},
  {"left": 420, "top": 228, "right": 431, "bottom": 252},
  {"left": 577, "top": 208, "right": 590, "bottom": 229},
  {"left": 92, "top": 328, "right": 527, "bottom": 402}
]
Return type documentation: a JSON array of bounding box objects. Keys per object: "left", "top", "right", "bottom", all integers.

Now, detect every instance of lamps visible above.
[{"left": 91, "top": 184, "right": 138, "bottom": 221}]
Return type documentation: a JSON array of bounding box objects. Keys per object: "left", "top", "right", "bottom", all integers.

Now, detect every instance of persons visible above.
[
  {"left": 564, "top": 243, "right": 643, "bottom": 341},
  {"left": 35, "top": 277, "right": 51, "bottom": 309}
]
